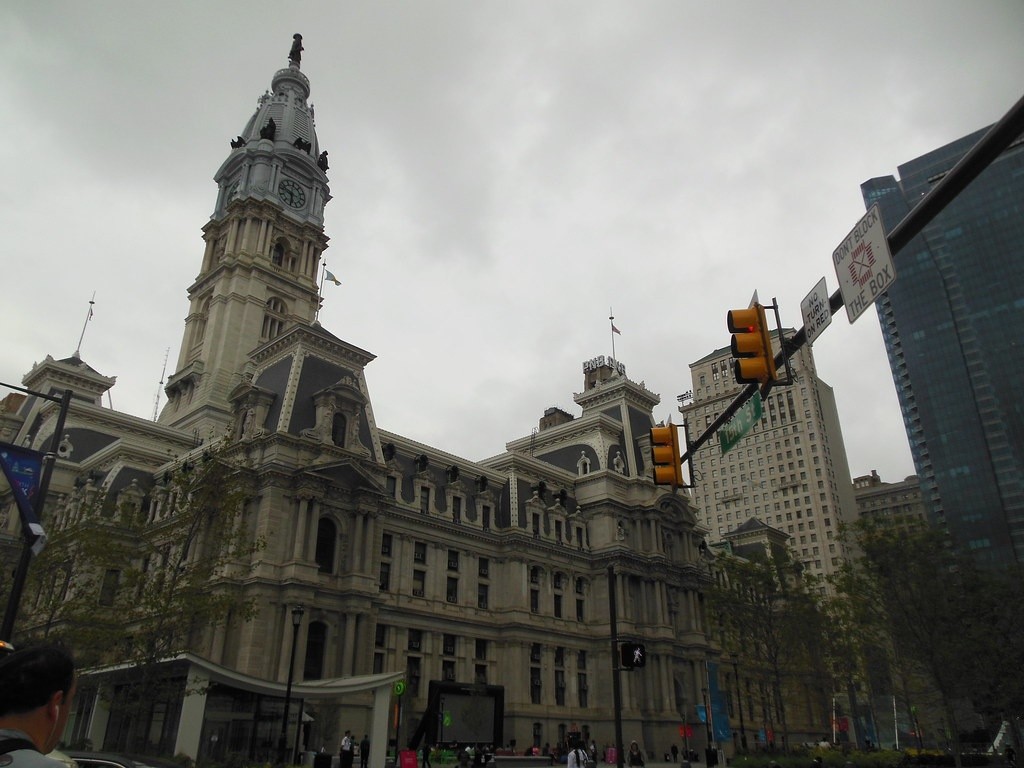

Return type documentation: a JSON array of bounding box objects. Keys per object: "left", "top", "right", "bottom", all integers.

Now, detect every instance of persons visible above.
[
  {"left": 339, "top": 730, "right": 695, "bottom": 768},
  {"left": 0, "top": 647, "right": 78, "bottom": 768},
  {"left": 567, "top": 736, "right": 589, "bottom": 768},
  {"left": 224, "top": 409, "right": 239, "bottom": 438},
  {"left": 321, "top": 744, "right": 328, "bottom": 754},
  {"left": 626, "top": 740, "right": 645, "bottom": 768},
  {"left": 819, "top": 737, "right": 832, "bottom": 749},
  {"left": 241, "top": 403, "right": 256, "bottom": 440},
  {"left": 323, "top": 402, "right": 338, "bottom": 444},
  {"left": 350, "top": 414, "right": 360, "bottom": 448}
]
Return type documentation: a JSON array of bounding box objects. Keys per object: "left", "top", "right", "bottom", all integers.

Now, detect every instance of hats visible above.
[{"left": 345, "top": 730, "right": 350, "bottom": 734}]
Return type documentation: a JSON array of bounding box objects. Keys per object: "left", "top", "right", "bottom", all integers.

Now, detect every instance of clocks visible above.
[{"left": 279, "top": 180, "right": 305, "bottom": 208}]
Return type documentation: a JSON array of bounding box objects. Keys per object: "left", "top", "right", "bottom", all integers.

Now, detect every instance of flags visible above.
[
  {"left": 89, "top": 308, "right": 93, "bottom": 321},
  {"left": 612, "top": 324, "right": 621, "bottom": 335},
  {"left": 325, "top": 270, "right": 341, "bottom": 286}
]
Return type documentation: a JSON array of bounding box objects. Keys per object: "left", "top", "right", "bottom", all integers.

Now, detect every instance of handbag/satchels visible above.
[{"left": 581, "top": 748, "right": 597, "bottom": 768}]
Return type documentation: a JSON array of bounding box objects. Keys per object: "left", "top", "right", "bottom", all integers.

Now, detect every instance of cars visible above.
[{"left": 60, "top": 751, "right": 184, "bottom": 768}]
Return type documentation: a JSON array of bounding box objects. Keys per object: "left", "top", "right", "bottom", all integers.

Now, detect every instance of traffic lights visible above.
[
  {"left": 650, "top": 421, "right": 682, "bottom": 485},
  {"left": 621, "top": 643, "right": 646, "bottom": 668},
  {"left": 727, "top": 304, "right": 777, "bottom": 385}
]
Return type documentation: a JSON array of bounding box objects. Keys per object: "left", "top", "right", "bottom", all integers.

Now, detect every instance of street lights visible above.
[
  {"left": 276, "top": 604, "right": 305, "bottom": 763},
  {"left": 701, "top": 687, "right": 712, "bottom": 751}
]
